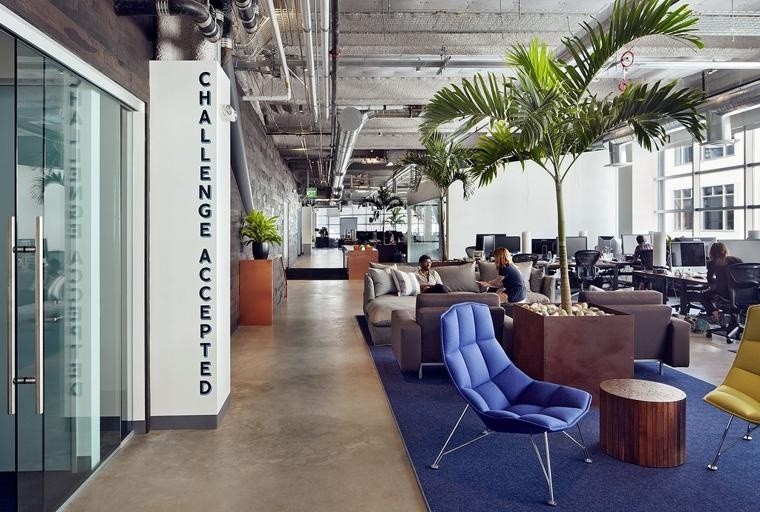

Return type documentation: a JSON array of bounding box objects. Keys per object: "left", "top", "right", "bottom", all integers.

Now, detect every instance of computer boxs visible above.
[{"left": 477, "top": 235, "right": 493, "bottom": 256}]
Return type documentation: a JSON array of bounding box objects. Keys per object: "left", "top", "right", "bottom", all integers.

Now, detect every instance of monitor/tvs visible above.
[
  {"left": 531, "top": 237, "right": 559, "bottom": 262},
  {"left": 555, "top": 236, "right": 590, "bottom": 261},
  {"left": 669, "top": 241, "right": 708, "bottom": 275},
  {"left": 621, "top": 233, "right": 654, "bottom": 262},
  {"left": 493, "top": 235, "right": 523, "bottom": 259}
]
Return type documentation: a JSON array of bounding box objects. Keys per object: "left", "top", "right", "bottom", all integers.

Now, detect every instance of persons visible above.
[
  {"left": 415, "top": 255, "right": 451, "bottom": 292},
  {"left": 697, "top": 243, "right": 743, "bottom": 319},
  {"left": 476, "top": 247, "right": 526, "bottom": 302},
  {"left": 630, "top": 236, "right": 653, "bottom": 283}
]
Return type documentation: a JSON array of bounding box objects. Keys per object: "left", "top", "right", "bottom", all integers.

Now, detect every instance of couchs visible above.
[
  {"left": 361, "top": 265, "right": 557, "bottom": 348},
  {"left": 574, "top": 287, "right": 692, "bottom": 375},
  {"left": 390, "top": 289, "right": 513, "bottom": 382}
]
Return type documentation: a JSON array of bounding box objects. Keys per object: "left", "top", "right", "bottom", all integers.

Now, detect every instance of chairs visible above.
[
  {"left": 566, "top": 249, "right": 602, "bottom": 291},
  {"left": 700, "top": 304, "right": 760, "bottom": 472},
  {"left": 511, "top": 253, "right": 538, "bottom": 266},
  {"left": 428, "top": 300, "right": 593, "bottom": 506},
  {"left": 704, "top": 262, "right": 760, "bottom": 343}
]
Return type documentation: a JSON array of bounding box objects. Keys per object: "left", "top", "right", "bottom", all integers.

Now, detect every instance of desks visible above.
[{"left": 630, "top": 265, "right": 713, "bottom": 315}]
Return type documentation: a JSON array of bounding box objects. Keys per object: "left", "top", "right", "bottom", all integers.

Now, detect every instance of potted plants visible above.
[
  {"left": 417, "top": 1, "right": 709, "bottom": 407},
  {"left": 354, "top": 183, "right": 406, "bottom": 263},
  {"left": 238, "top": 210, "right": 282, "bottom": 261}
]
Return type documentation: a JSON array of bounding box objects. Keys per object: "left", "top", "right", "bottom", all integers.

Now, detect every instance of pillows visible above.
[
  {"left": 434, "top": 264, "right": 484, "bottom": 294},
  {"left": 477, "top": 260, "right": 498, "bottom": 280},
  {"left": 391, "top": 266, "right": 426, "bottom": 296},
  {"left": 528, "top": 266, "right": 545, "bottom": 292},
  {"left": 511, "top": 261, "right": 534, "bottom": 291},
  {"left": 368, "top": 265, "right": 396, "bottom": 296}
]
